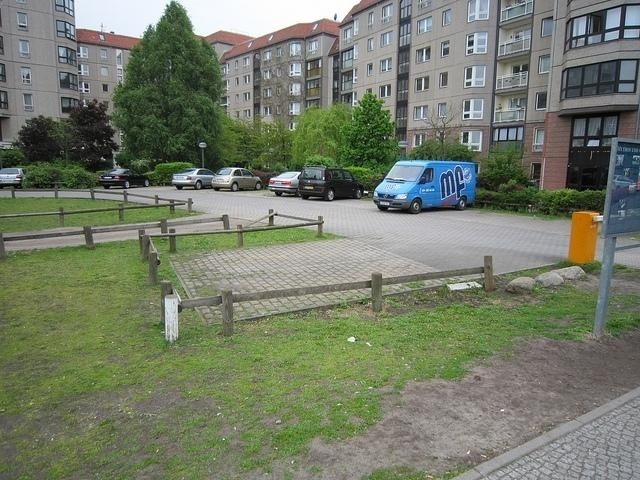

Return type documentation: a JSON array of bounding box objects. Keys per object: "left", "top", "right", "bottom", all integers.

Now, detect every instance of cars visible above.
[
  {"left": 212, "top": 166, "right": 263, "bottom": 191},
  {"left": 172, "top": 168, "right": 217, "bottom": 190},
  {"left": 97, "top": 168, "right": 151, "bottom": 189},
  {"left": 0, "top": 167, "right": 26, "bottom": 190},
  {"left": 268, "top": 171, "right": 302, "bottom": 197}
]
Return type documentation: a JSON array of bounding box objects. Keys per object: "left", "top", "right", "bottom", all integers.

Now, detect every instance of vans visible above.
[{"left": 298, "top": 163, "right": 365, "bottom": 199}]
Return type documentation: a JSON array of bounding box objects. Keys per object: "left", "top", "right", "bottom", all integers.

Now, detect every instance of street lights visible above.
[{"left": 198, "top": 142, "right": 207, "bottom": 168}]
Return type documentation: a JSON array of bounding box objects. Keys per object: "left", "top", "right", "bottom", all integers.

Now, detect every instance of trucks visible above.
[{"left": 373, "top": 159, "right": 477, "bottom": 212}]
